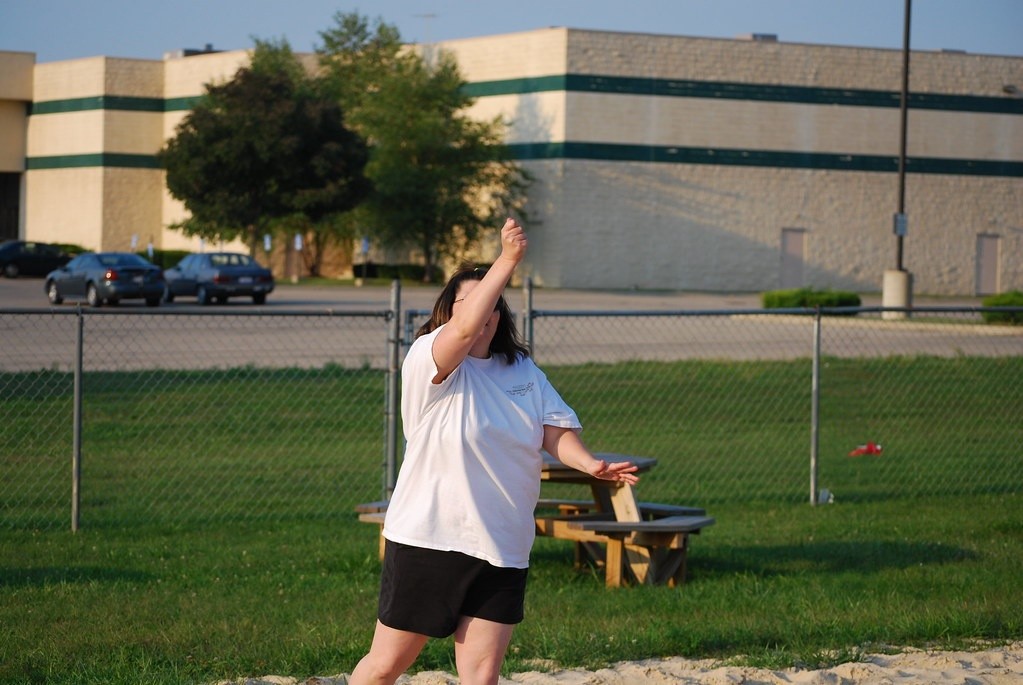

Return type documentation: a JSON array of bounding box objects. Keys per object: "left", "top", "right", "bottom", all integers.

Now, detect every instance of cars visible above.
[
  {"left": 0, "top": 240, "right": 94, "bottom": 278},
  {"left": 164, "top": 253, "right": 276, "bottom": 308},
  {"left": 44, "top": 250, "right": 165, "bottom": 307}
]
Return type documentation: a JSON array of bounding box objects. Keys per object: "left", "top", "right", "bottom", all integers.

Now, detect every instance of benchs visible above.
[{"left": 356, "top": 498, "right": 715, "bottom": 591}]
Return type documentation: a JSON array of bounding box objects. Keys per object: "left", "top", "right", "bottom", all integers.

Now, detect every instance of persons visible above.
[{"left": 347, "top": 217, "right": 641, "bottom": 685}]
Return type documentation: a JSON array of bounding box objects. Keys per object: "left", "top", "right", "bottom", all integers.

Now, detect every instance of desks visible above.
[{"left": 540, "top": 451, "right": 659, "bottom": 588}]
